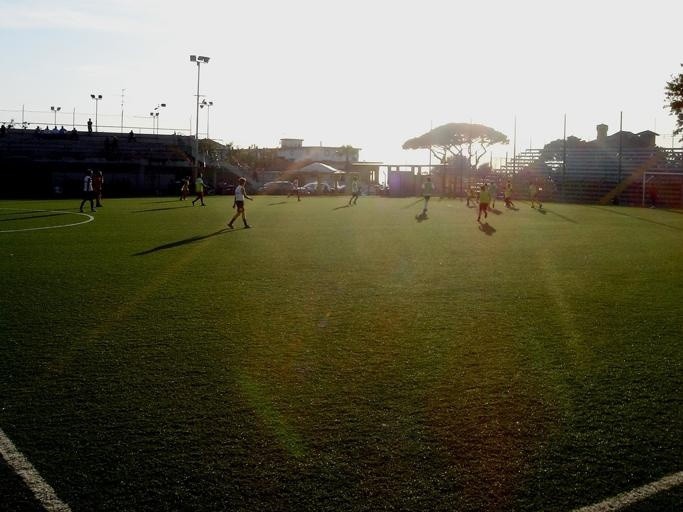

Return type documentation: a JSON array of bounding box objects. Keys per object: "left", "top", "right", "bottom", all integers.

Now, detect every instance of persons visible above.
[
  {"left": 527, "top": 181, "right": 542, "bottom": 209},
  {"left": 127, "top": 131, "right": 136, "bottom": 143},
  {"left": 419, "top": 177, "right": 435, "bottom": 211},
  {"left": 286, "top": 175, "right": 302, "bottom": 201},
  {"left": 178, "top": 176, "right": 191, "bottom": 201},
  {"left": 190, "top": 171, "right": 207, "bottom": 206},
  {"left": 474, "top": 185, "right": 489, "bottom": 222},
  {"left": 33, "top": 124, "right": 78, "bottom": 134},
  {"left": 502, "top": 179, "right": 515, "bottom": 209},
  {"left": 647, "top": 182, "right": 658, "bottom": 209},
  {"left": 93, "top": 170, "right": 103, "bottom": 208},
  {"left": 86, "top": 118, "right": 92, "bottom": 132},
  {"left": 347, "top": 175, "right": 359, "bottom": 207},
  {"left": 225, "top": 177, "right": 254, "bottom": 229},
  {"left": 465, "top": 185, "right": 473, "bottom": 207},
  {"left": 78, "top": 168, "right": 96, "bottom": 213},
  {"left": 488, "top": 182, "right": 497, "bottom": 209}
]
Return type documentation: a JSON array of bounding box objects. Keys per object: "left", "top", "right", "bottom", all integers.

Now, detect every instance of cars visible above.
[{"left": 257, "top": 180, "right": 334, "bottom": 195}]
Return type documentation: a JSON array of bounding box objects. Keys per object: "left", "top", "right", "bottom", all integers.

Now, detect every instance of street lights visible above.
[
  {"left": 190, "top": 55, "right": 210, "bottom": 195},
  {"left": 0, "top": 88, "right": 213, "bottom": 140}
]
